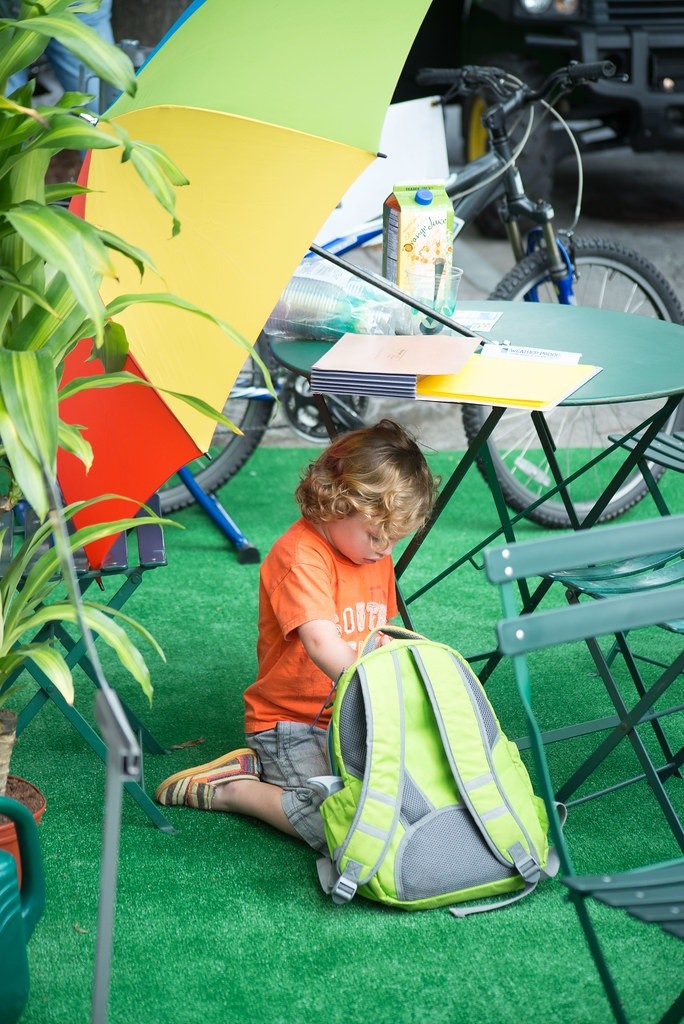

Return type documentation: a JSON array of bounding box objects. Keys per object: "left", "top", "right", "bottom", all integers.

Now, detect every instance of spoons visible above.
[{"left": 419, "top": 258, "right": 444, "bottom": 335}]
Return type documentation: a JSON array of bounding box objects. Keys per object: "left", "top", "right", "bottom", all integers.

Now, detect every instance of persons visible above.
[{"left": 155, "top": 418, "right": 433, "bottom": 852}]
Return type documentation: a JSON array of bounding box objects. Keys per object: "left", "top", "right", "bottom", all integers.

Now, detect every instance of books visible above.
[{"left": 310, "top": 332, "right": 483, "bottom": 399}]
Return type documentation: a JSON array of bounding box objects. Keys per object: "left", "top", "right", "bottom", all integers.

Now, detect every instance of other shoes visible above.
[{"left": 155, "top": 747, "right": 262, "bottom": 810}]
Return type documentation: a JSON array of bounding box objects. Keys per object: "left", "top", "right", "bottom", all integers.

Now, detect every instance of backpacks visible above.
[{"left": 317, "top": 625, "right": 566, "bottom": 918}]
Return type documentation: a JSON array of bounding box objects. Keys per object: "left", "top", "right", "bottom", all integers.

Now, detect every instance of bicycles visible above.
[{"left": 160, "top": 59, "right": 684, "bottom": 529}]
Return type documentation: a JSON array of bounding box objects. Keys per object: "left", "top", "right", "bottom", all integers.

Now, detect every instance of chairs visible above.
[{"left": 482, "top": 514, "right": 684, "bottom": 1024}]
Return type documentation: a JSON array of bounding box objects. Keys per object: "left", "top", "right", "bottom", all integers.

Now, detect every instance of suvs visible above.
[{"left": 394, "top": 0, "right": 684, "bottom": 236}]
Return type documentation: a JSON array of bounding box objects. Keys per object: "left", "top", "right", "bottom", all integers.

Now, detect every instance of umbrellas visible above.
[{"left": 57, "top": 0, "right": 490, "bottom": 568}]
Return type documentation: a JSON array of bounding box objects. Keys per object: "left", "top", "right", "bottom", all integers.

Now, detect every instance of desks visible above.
[{"left": 268, "top": 300, "right": 684, "bottom": 790}]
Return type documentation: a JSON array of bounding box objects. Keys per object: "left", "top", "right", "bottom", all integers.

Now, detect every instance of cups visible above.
[
  {"left": 263, "top": 259, "right": 421, "bottom": 341},
  {"left": 407, "top": 264, "right": 463, "bottom": 336}
]
